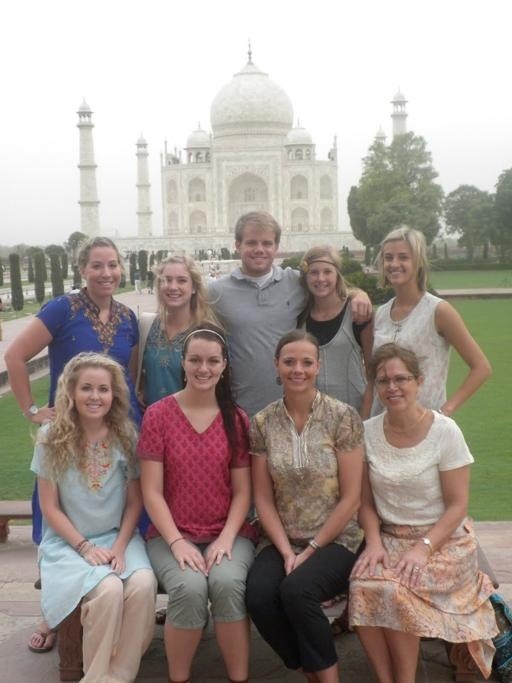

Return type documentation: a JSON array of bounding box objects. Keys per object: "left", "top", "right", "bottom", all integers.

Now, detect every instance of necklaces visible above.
[{"left": 386, "top": 408, "right": 425, "bottom": 434}]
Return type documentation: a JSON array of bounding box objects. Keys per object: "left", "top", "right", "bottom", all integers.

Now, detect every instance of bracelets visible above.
[
  {"left": 169, "top": 536, "right": 185, "bottom": 551},
  {"left": 75, "top": 539, "right": 97, "bottom": 558},
  {"left": 309, "top": 539, "right": 320, "bottom": 552},
  {"left": 439, "top": 410, "right": 445, "bottom": 415},
  {"left": 420, "top": 537, "right": 435, "bottom": 555}
]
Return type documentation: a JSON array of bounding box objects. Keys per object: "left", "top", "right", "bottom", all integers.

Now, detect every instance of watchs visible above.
[{"left": 20, "top": 402, "right": 38, "bottom": 419}]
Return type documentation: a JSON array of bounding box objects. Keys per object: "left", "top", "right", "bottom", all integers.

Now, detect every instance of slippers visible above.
[{"left": 28, "top": 621, "right": 58, "bottom": 653}]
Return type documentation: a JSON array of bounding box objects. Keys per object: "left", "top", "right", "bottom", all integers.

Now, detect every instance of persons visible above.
[
  {"left": 374, "top": 224, "right": 492, "bottom": 418},
  {"left": 132, "top": 264, "right": 156, "bottom": 296},
  {"left": 130, "top": 253, "right": 233, "bottom": 627},
  {"left": 197, "top": 247, "right": 221, "bottom": 280},
  {"left": 347, "top": 341, "right": 500, "bottom": 682},
  {"left": 3, "top": 237, "right": 147, "bottom": 653},
  {"left": 29, "top": 350, "right": 157, "bottom": 682},
  {"left": 298, "top": 244, "right": 374, "bottom": 639},
  {"left": 247, "top": 330, "right": 365, "bottom": 683},
  {"left": 136, "top": 322, "right": 258, "bottom": 683},
  {"left": 200, "top": 211, "right": 374, "bottom": 414}
]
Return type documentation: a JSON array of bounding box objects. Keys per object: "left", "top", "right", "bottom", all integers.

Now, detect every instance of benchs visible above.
[{"left": 33, "top": 568, "right": 491, "bottom": 680}]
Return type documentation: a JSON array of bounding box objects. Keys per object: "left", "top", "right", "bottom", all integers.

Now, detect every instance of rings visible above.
[
  {"left": 414, "top": 566, "right": 420, "bottom": 573},
  {"left": 220, "top": 549, "right": 225, "bottom": 554}
]
